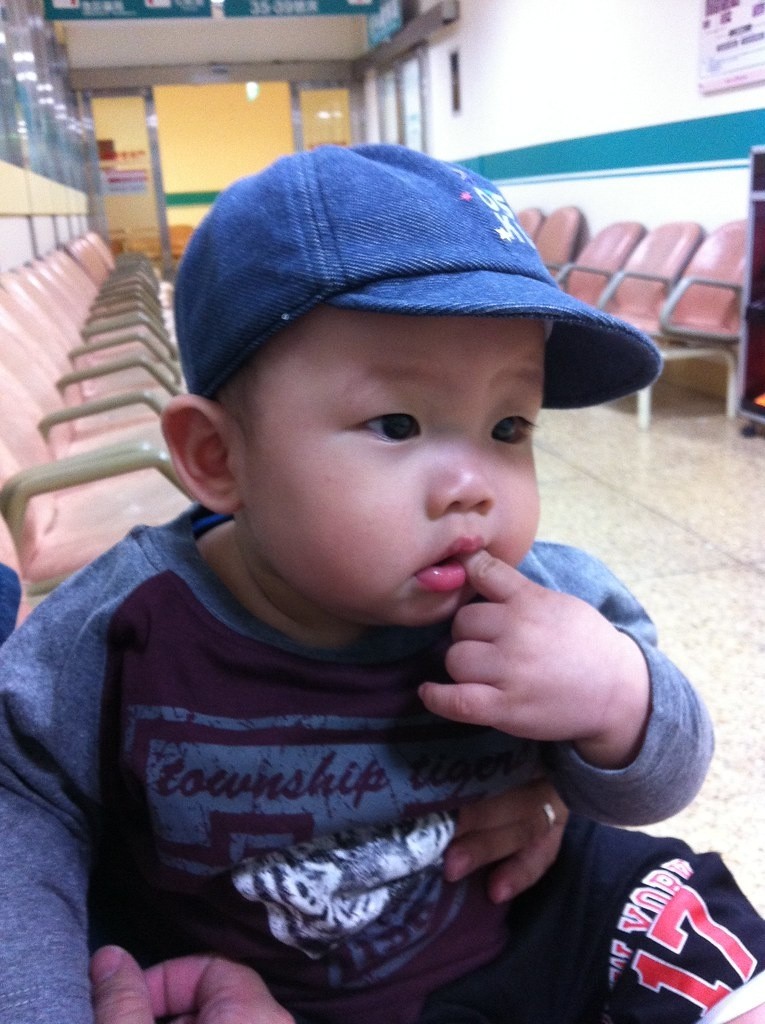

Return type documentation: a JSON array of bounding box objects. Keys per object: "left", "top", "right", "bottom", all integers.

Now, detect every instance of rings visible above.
[{"left": 543, "top": 803, "right": 556, "bottom": 824}]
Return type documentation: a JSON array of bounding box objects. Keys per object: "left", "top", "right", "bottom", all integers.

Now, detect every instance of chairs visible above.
[
  {"left": 0, "top": 230, "right": 194, "bottom": 625},
  {"left": 525, "top": 205, "right": 584, "bottom": 272},
  {"left": 626, "top": 220, "right": 746, "bottom": 428},
  {"left": 546, "top": 221, "right": 647, "bottom": 306},
  {"left": 517, "top": 205, "right": 543, "bottom": 239},
  {"left": 588, "top": 220, "right": 706, "bottom": 344}
]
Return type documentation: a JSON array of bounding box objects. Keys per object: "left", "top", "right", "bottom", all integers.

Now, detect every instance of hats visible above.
[{"left": 174, "top": 146, "right": 663, "bottom": 410}]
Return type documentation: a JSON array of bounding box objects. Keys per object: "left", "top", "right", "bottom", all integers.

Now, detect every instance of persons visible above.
[{"left": 0, "top": 143, "right": 765, "bottom": 1024}]
